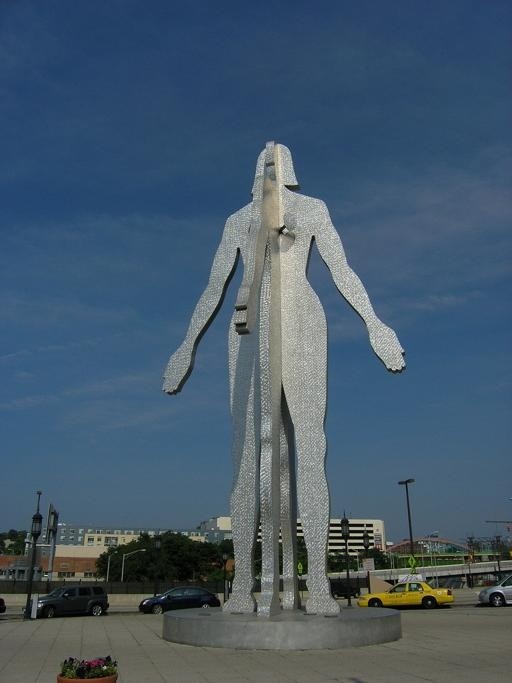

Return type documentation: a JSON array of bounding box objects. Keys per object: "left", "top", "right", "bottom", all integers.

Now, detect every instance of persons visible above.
[{"left": 162, "top": 143, "right": 408, "bottom": 616}]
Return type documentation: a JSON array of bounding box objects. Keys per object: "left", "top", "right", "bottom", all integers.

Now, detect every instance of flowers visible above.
[{"left": 60, "top": 654, "right": 119, "bottom": 679}]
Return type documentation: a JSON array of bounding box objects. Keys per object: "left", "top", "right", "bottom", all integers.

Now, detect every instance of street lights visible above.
[
  {"left": 398, "top": 479, "right": 416, "bottom": 572},
  {"left": 340, "top": 512, "right": 352, "bottom": 605},
  {"left": 22, "top": 490, "right": 43, "bottom": 619},
  {"left": 363, "top": 530, "right": 370, "bottom": 557},
  {"left": 106, "top": 550, "right": 119, "bottom": 584},
  {"left": 121, "top": 548, "right": 147, "bottom": 583},
  {"left": 429, "top": 530, "right": 439, "bottom": 564}
]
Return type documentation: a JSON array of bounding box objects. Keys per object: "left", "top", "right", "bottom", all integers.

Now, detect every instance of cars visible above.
[
  {"left": 358, "top": 580, "right": 455, "bottom": 609},
  {"left": 479, "top": 573, "right": 512, "bottom": 605},
  {"left": 139, "top": 585, "right": 220, "bottom": 614}
]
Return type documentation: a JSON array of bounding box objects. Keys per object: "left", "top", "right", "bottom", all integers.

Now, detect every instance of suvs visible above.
[{"left": 24, "top": 584, "right": 109, "bottom": 618}]
